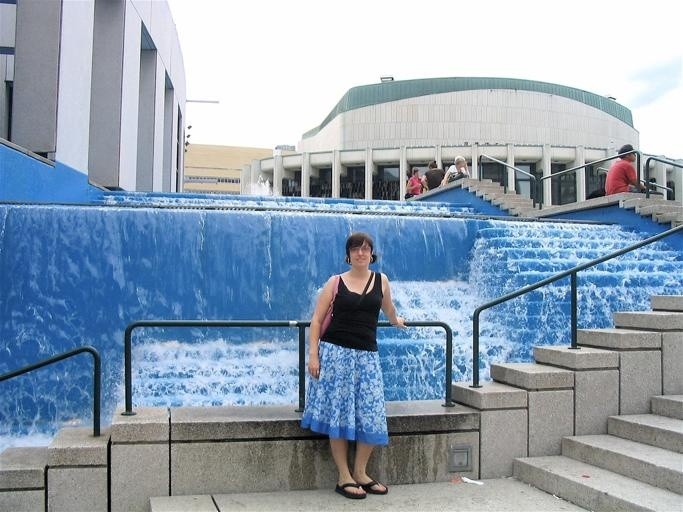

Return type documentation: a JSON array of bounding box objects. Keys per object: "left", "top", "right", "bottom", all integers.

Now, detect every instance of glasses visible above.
[{"left": 347, "top": 246, "right": 371, "bottom": 253}]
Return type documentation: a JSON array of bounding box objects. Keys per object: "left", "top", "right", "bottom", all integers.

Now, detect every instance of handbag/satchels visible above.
[{"left": 320, "top": 275, "right": 340, "bottom": 338}]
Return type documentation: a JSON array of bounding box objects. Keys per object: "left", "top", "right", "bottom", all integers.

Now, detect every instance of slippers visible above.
[
  {"left": 350, "top": 473, "right": 389, "bottom": 495},
  {"left": 335, "top": 481, "right": 366, "bottom": 500}
]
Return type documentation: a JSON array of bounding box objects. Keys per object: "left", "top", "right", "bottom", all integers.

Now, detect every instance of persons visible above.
[
  {"left": 439, "top": 155, "right": 469, "bottom": 185},
  {"left": 403, "top": 167, "right": 422, "bottom": 195},
  {"left": 300, "top": 233, "right": 407, "bottom": 498},
  {"left": 421, "top": 160, "right": 444, "bottom": 190},
  {"left": 604, "top": 143, "right": 664, "bottom": 195}
]
